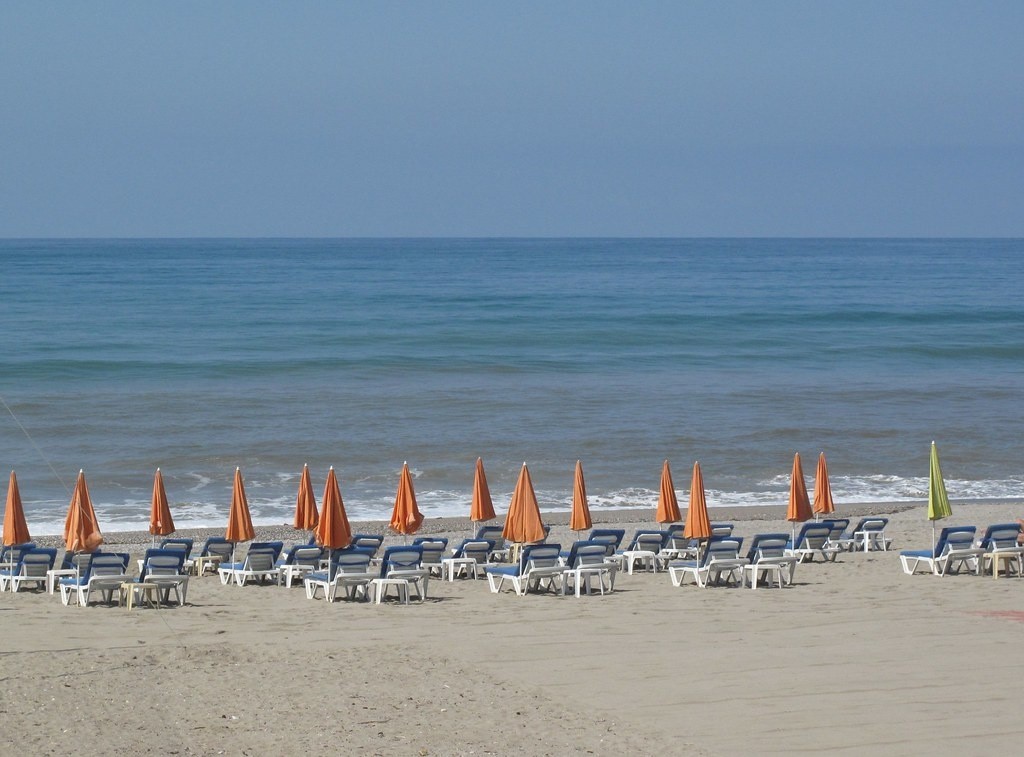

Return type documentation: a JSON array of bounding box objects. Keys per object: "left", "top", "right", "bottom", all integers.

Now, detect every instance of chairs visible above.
[{"left": 0, "top": 517, "right": 1024, "bottom": 610}]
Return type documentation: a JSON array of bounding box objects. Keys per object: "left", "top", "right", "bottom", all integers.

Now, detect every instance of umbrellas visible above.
[
  {"left": 149, "top": 466, "right": 175, "bottom": 550},
  {"left": 314, "top": 466, "right": 351, "bottom": 581},
  {"left": 390, "top": 461, "right": 424, "bottom": 546},
  {"left": 63, "top": 469, "right": 102, "bottom": 607},
  {"left": 501, "top": 462, "right": 545, "bottom": 592},
  {"left": 787, "top": 452, "right": 812, "bottom": 551},
  {"left": 570, "top": 460, "right": 592, "bottom": 542},
  {"left": 470, "top": 457, "right": 496, "bottom": 539},
  {"left": 684, "top": 461, "right": 712, "bottom": 571},
  {"left": 293, "top": 463, "right": 319, "bottom": 546},
  {"left": 813, "top": 452, "right": 835, "bottom": 523},
  {"left": 226, "top": 466, "right": 256, "bottom": 584},
  {"left": 928, "top": 441, "right": 952, "bottom": 558},
  {"left": 2, "top": 470, "right": 31, "bottom": 592},
  {"left": 656, "top": 460, "right": 682, "bottom": 531}
]
